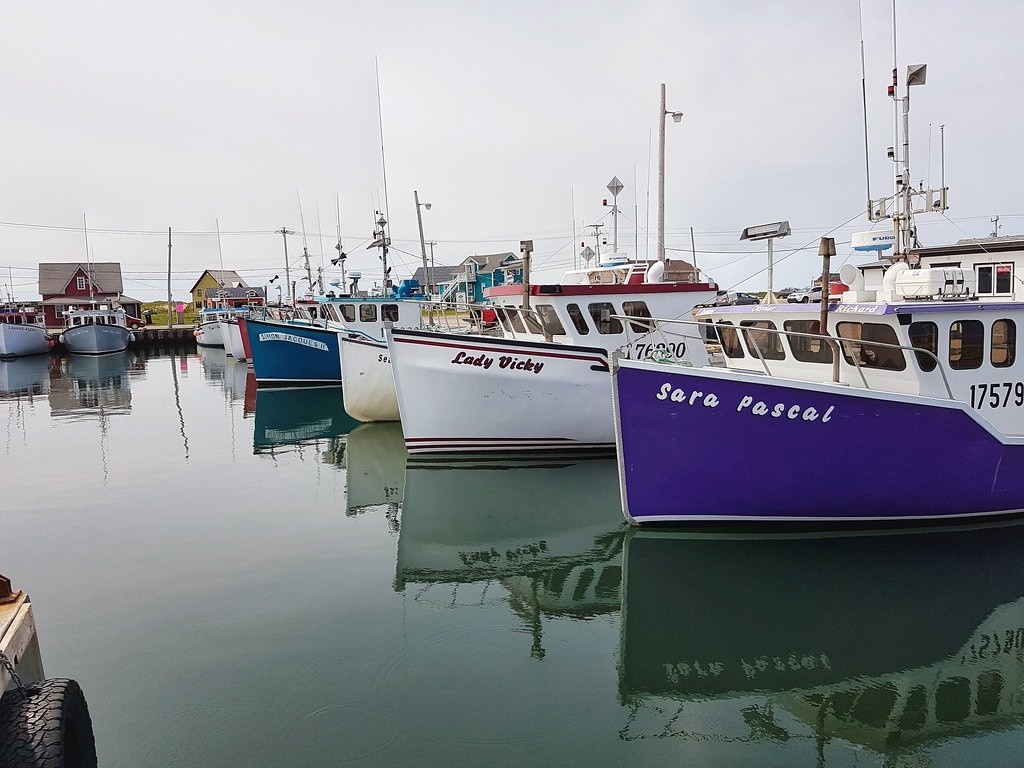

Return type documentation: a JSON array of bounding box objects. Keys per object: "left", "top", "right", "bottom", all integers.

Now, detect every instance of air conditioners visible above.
[{"left": 468, "top": 296, "right": 475, "bottom": 301}]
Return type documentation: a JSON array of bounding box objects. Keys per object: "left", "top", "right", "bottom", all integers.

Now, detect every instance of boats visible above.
[
  {"left": 383, "top": 281, "right": 721, "bottom": 460},
  {"left": 608, "top": 1, "right": 1023, "bottom": 524},
  {"left": 346, "top": 421, "right": 407, "bottom": 517},
  {"left": 0, "top": 353, "right": 49, "bottom": 394},
  {"left": 617, "top": 525, "right": 1024, "bottom": 767},
  {"left": 195, "top": 225, "right": 430, "bottom": 384},
  {"left": 254, "top": 385, "right": 362, "bottom": 454},
  {"left": 336, "top": 330, "right": 400, "bottom": 421},
  {"left": 0, "top": 322, "right": 55, "bottom": 358},
  {"left": 392, "top": 462, "right": 630, "bottom": 660},
  {"left": 197, "top": 344, "right": 257, "bottom": 418}
]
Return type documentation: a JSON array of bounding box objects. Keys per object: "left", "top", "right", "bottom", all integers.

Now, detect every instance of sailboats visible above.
[
  {"left": 60, "top": 352, "right": 137, "bottom": 479},
  {"left": 58, "top": 211, "right": 135, "bottom": 355}
]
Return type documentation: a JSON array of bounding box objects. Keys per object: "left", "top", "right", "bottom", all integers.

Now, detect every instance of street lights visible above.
[
  {"left": 657, "top": 84, "right": 684, "bottom": 262},
  {"left": 413, "top": 191, "right": 431, "bottom": 297}
]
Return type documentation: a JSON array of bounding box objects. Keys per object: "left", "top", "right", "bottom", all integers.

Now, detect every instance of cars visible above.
[
  {"left": 787, "top": 286, "right": 822, "bottom": 304},
  {"left": 716, "top": 290, "right": 761, "bottom": 306},
  {"left": 125, "top": 314, "right": 145, "bottom": 330}
]
